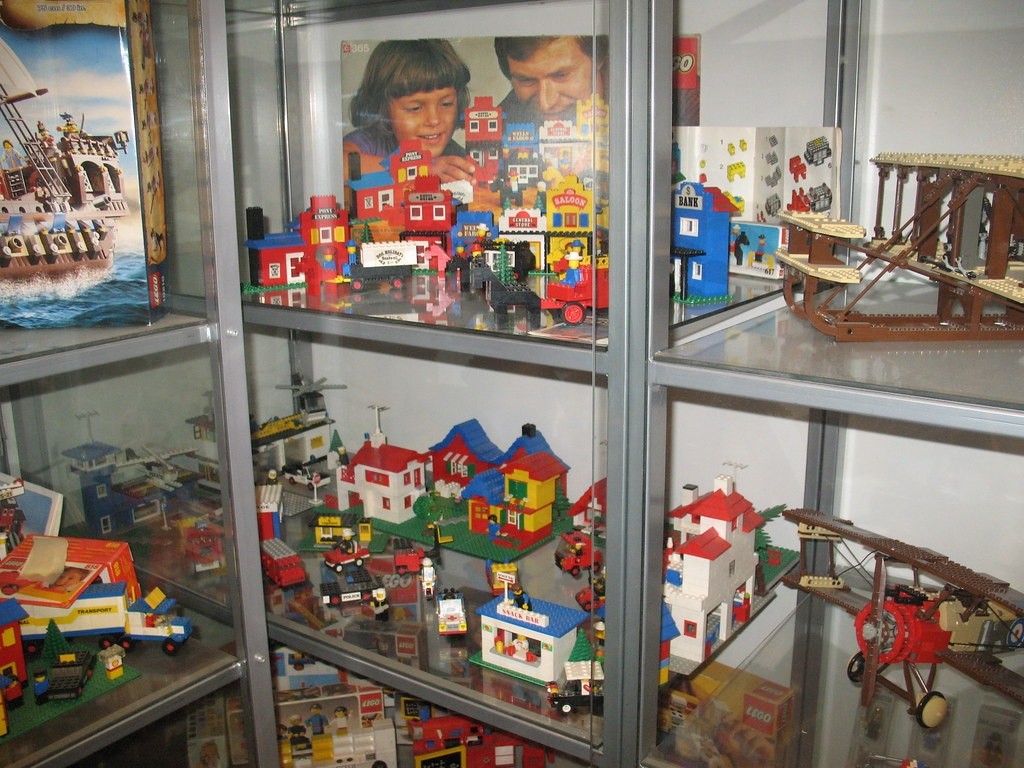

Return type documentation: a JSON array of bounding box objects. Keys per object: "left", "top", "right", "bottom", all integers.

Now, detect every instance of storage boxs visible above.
[
  {"left": 0, "top": 0, "right": 173, "bottom": 329},
  {"left": 185, "top": 552, "right": 430, "bottom": 768},
  {"left": 671, "top": 126, "right": 841, "bottom": 279},
  {"left": 678, "top": 663, "right": 794, "bottom": 768}
]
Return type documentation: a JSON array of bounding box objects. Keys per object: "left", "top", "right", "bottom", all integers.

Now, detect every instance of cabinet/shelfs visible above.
[
  {"left": 0, "top": 0, "right": 281, "bottom": 768},
  {"left": 61, "top": 0, "right": 629, "bottom": 768},
  {"left": 622, "top": 0, "right": 1024, "bottom": 768}
]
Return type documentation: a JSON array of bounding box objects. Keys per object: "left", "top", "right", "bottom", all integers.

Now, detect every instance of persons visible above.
[
  {"left": 494, "top": 37, "right": 609, "bottom": 137},
  {"left": 418, "top": 558, "right": 437, "bottom": 586},
  {"left": 278, "top": 704, "right": 354, "bottom": 749},
  {"left": 454, "top": 241, "right": 468, "bottom": 259},
  {"left": 266, "top": 470, "right": 278, "bottom": 486},
  {"left": 562, "top": 252, "right": 582, "bottom": 287},
  {"left": 509, "top": 635, "right": 529, "bottom": 657},
  {"left": 370, "top": 588, "right": 390, "bottom": 621},
  {"left": 45, "top": 568, "right": 89, "bottom": 593},
  {"left": 571, "top": 240, "right": 584, "bottom": 253},
  {"left": 321, "top": 248, "right": 336, "bottom": 270},
  {"left": 34, "top": 670, "right": 50, "bottom": 705},
  {"left": 345, "top": 240, "right": 358, "bottom": 265},
  {"left": 5, "top": 675, "right": 25, "bottom": 710},
  {"left": 335, "top": 447, "right": 349, "bottom": 467},
  {"left": 343, "top": 40, "right": 477, "bottom": 204},
  {"left": 486, "top": 514, "right": 500, "bottom": 541},
  {"left": 342, "top": 528, "right": 355, "bottom": 552},
  {"left": 475, "top": 223, "right": 489, "bottom": 243},
  {"left": 146, "top": 612, "right": 165, "bottom": 627},
  {"left": 509, "top": 582, "right": 532, "bottom": 611}
]
